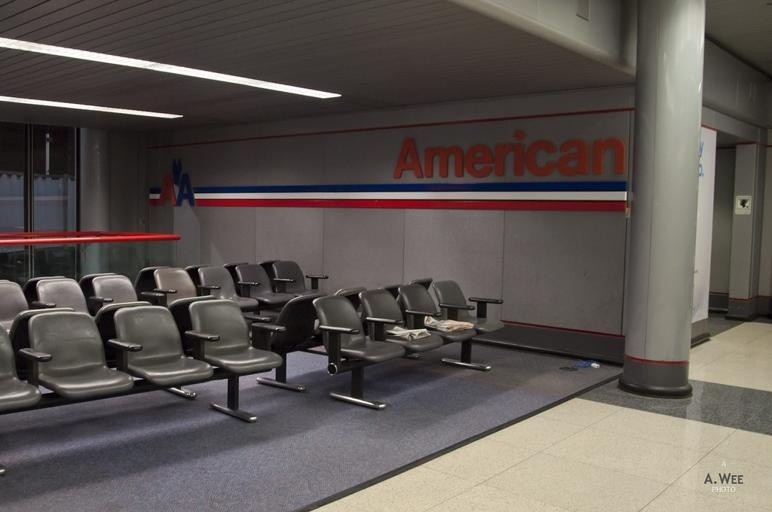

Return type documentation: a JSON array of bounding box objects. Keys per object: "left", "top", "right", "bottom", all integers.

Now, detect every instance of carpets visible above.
[{"left": 0, "top": 336, "right": 624, "bottom": 512}]
